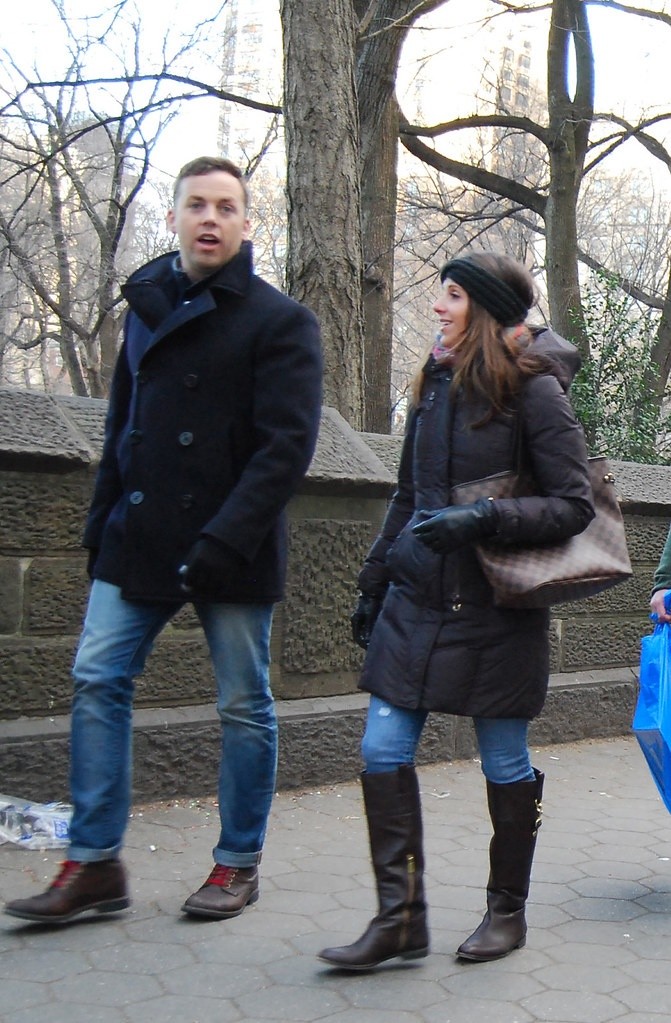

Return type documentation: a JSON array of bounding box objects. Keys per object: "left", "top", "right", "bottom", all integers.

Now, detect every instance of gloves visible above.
[
  {"left": 87, "top": 548, "right": 96, "bottom": 580},
  {"left": 351, "top": 590, "right": 387, "bottom": 651},
  {"left": 412, "top": 504, "right": 485, "bottom": 555},
  {"left": 174, "top": 539, "right": 242, "bottom": 600}
]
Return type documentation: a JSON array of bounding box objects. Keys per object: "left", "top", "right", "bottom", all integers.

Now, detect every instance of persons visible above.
[
  {"left": 313, "top": 250, "right": 633, "bottom": 972},
  {"left": 0, "top": 156, "right": 324, "bottom": 920},
  {"left": 650, "top": 524, "right": 671, "bottom": 624}
]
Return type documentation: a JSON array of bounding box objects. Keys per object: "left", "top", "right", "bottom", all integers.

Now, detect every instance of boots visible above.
[
  {"left": 316, "top": 761, "right": 431, "bottom": 970},
  {"left": 456, "top": 766, "right": 545, "bottom": 961}
]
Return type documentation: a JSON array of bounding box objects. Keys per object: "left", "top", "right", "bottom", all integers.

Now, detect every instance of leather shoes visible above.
[
  {"left": 180, "top": 864, "right": 259, "bottom": 917},
  {"left": 5, "top": 861, "right": 129, "bottom": 921}
]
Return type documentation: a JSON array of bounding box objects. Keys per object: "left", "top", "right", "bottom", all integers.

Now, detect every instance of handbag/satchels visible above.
[
  {"left": 632, "top": 590, "right": 671, "bottom": 815},
  {"left": 451, "top": 456, "right": 633, "bottom": 607}
]
